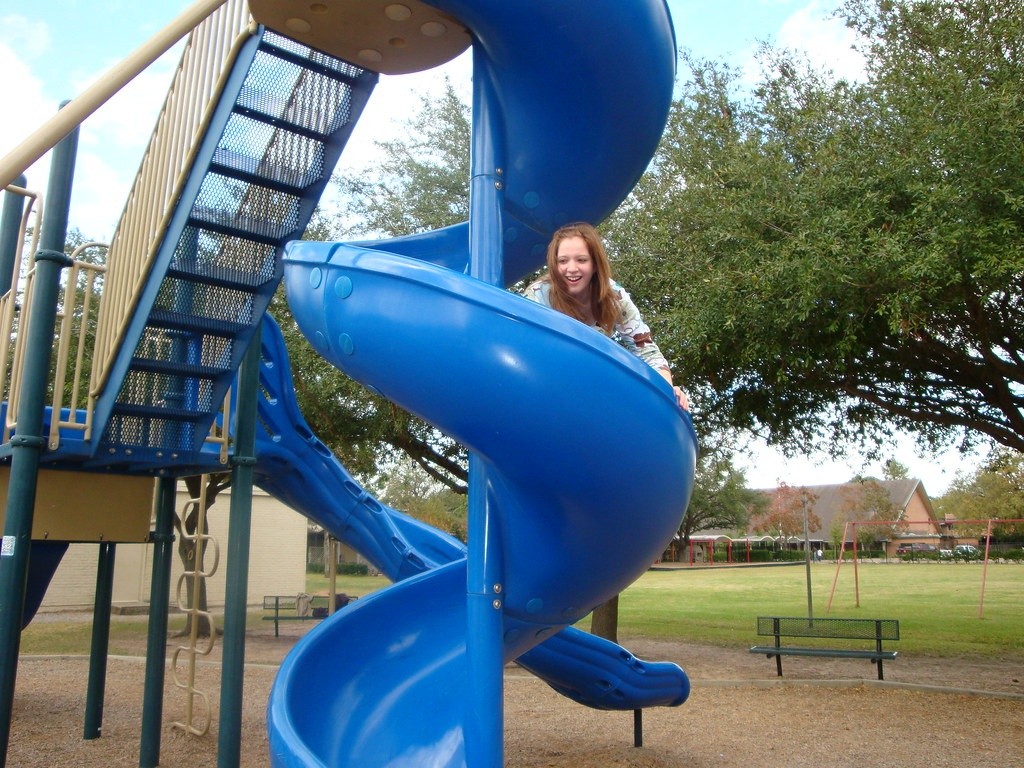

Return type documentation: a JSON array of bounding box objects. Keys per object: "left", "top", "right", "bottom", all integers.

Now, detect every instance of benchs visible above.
[
  {"left": 749, "top": 616, "right": 899, "bottom": 680},
  {"left": 261, "top": 595, "right": 359, "bottom": 639}
]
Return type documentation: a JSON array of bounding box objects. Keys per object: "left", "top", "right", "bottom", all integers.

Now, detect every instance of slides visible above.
[
  {"left": 266, "top": 0, "right": 701, "bottom": 768},
  {"left": 255, "top": 310, "right": 690, "bottom": 712}
]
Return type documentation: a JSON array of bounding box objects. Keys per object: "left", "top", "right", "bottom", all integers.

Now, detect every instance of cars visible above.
[{"left": 897, "top": 543, "right": 979, "bottom": 558}]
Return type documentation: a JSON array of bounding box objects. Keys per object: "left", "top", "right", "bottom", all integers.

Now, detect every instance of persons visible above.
[
  {"left": 817, "top": 549, "right": 823, "bottom": 561},
  {"left": 528, "top": 220, "right": 693, "bottom": 412}
]
